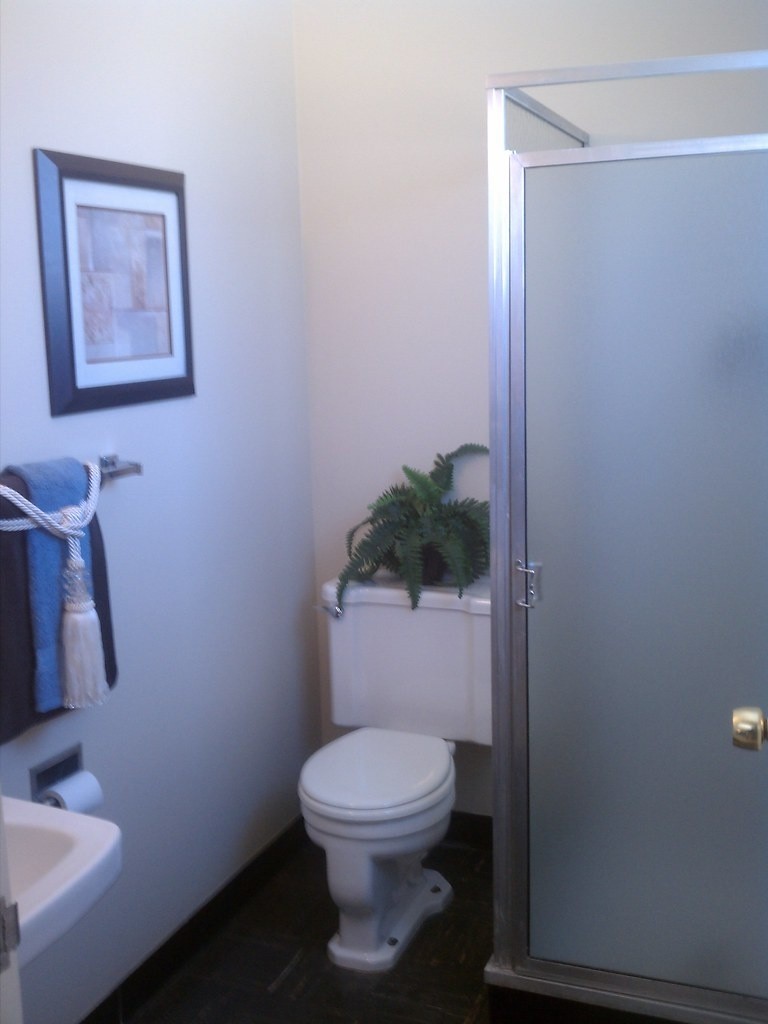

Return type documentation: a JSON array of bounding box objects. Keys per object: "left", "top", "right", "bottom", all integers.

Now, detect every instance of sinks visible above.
[{"left": 3, "top": 794, "right": 126, "bottom": 984}]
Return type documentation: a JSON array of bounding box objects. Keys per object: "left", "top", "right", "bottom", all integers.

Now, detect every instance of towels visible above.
[
  {"left": 0, "top": 468, "right": 120, "bottom": 745},
  {"left": 4, "top": 454, "right": 110, "bottom": 712}
]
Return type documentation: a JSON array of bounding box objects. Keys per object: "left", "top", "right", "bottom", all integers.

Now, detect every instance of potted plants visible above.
[{"left": 333, "top": 440, "right": 492, "bottom": 617}]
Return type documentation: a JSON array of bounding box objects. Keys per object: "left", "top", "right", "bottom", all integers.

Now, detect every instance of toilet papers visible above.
[{"left": 41, "top": 771, "right": 109, "bottom": 814}]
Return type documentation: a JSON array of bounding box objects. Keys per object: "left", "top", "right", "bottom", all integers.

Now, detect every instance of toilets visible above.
[{"left": 294, "top": 572, "right": 496, "bottom": 973}]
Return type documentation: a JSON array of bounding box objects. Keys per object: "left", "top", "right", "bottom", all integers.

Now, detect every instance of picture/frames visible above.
[{"left": 31, "top": 149, "right": 199, "bottom": 420}]
